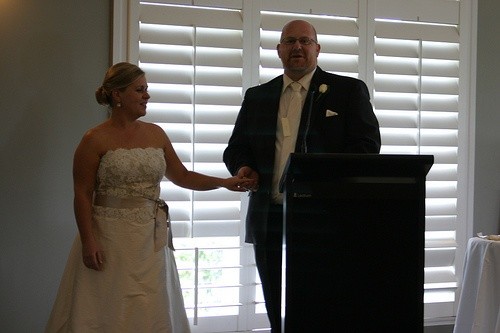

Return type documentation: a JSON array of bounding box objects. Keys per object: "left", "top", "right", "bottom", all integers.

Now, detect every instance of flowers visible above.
[{"left": 315, "top": 84, "right": 327, "bottom": 102}]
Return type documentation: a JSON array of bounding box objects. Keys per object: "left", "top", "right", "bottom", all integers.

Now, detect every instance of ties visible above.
[{"left": 278, "top": 83, "right": 302, "bottom": 177}]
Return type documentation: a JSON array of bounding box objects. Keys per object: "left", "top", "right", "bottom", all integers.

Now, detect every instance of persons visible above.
[
  {"left": 46, "top": 62, "right": 254, "bottom": 333},
  {"left": 222, "top": 20, "right": 381, "bottom": 333}
]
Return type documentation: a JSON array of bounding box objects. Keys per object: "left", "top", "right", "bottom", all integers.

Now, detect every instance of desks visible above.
[{"left": 452, "top": 235, "right": 500, "bottom": 333}]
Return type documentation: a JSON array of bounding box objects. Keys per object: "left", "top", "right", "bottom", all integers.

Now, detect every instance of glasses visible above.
[{"left": 280, "top": 36, "right": 318, "bottom": 45}]
